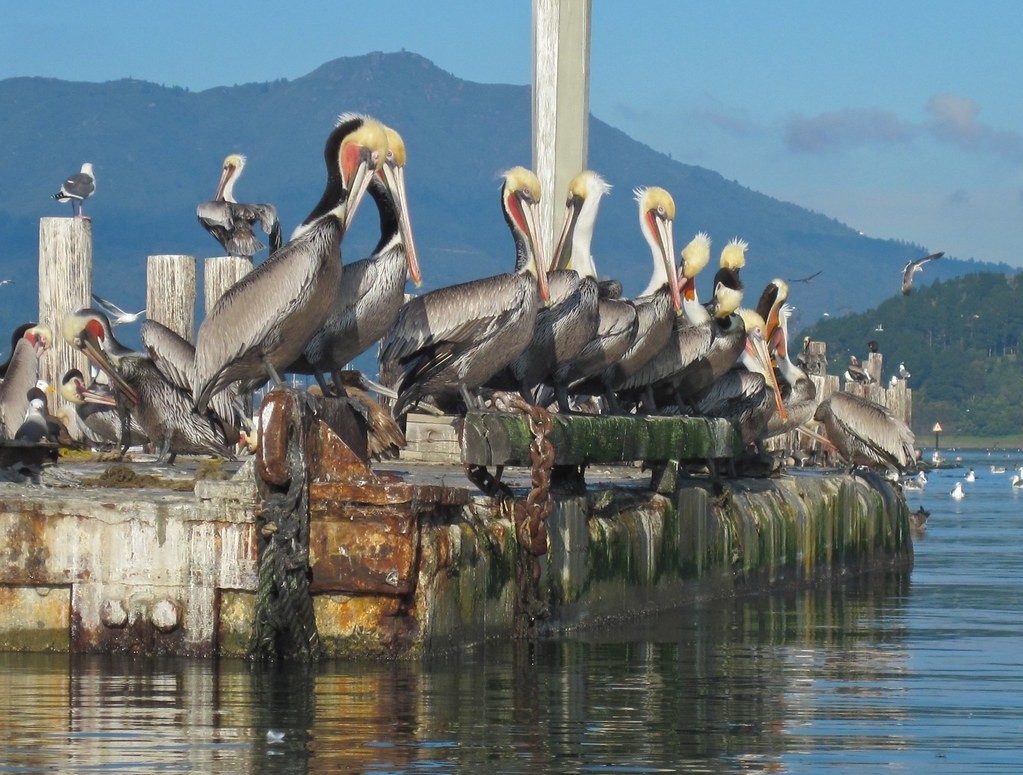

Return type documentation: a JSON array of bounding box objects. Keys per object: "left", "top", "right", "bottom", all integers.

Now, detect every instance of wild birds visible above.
[{"left": 0, "top": 112, "right": 1023, "bottom": 516}]
